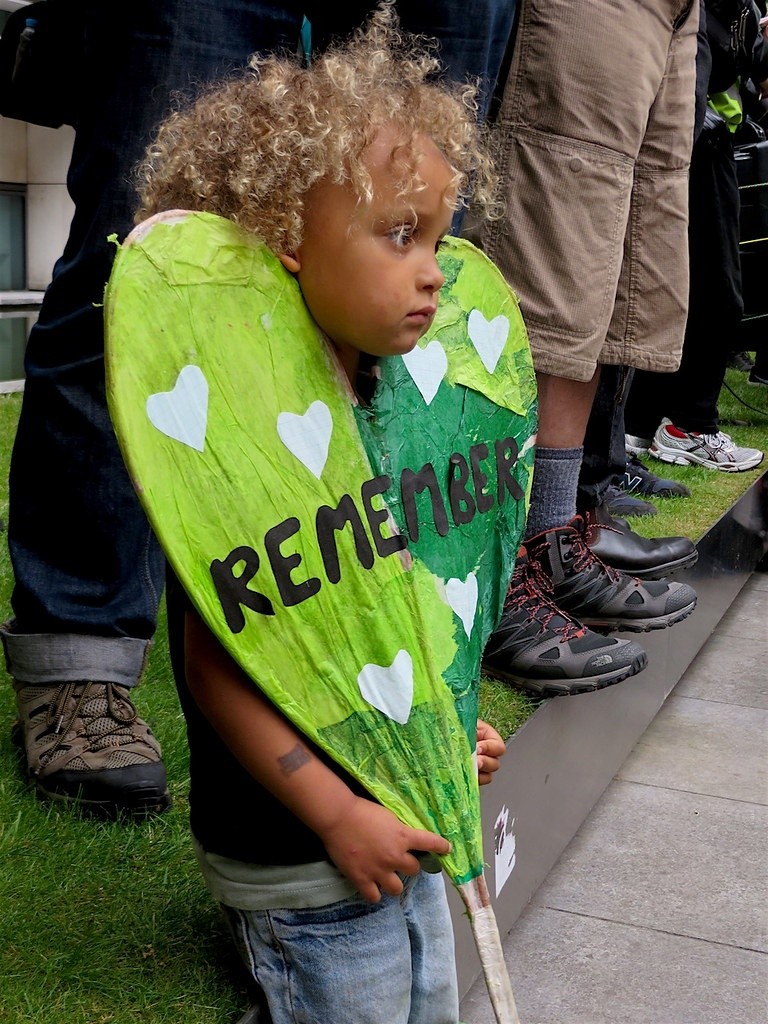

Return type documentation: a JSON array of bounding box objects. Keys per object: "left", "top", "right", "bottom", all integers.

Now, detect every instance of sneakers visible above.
[
  {"left": 522, "top": 514, "right": 697, "bottom": 631},
  {"left": 12, "top": 672, "right": 171, "bottom": 823},
  {"left": 648, "top": 416, "right": 764, "bottom": 472},
  {"left": 612, "top": 450, "right": 692, "bottom": 500},
  {"left": 477, "top": 544, "right": 649, "bottom": 700},
  {"left": 599, "top": 482, "right": 658, "bottom": 515},
  {"left": 623, "top": 432, "right": 651, "bottom": 455}
]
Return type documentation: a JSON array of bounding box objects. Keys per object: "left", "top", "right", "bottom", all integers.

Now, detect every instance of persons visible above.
[
  {"left": 576, "top": 0, "right": 767, "bottom": 582},
  {"left": 127, "top": 22, "right": 506, "bottom": 1023},
  {"left": 2, "top": 1, "right": 520, "bottom": 824},
  {"left": 454, "top": 0, "right": 702, "bottom": 697}
]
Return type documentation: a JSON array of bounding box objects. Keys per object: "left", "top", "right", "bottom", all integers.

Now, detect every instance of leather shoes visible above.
[{"left": 578, "top": 496, "right": 697, "bottom": 576}]
